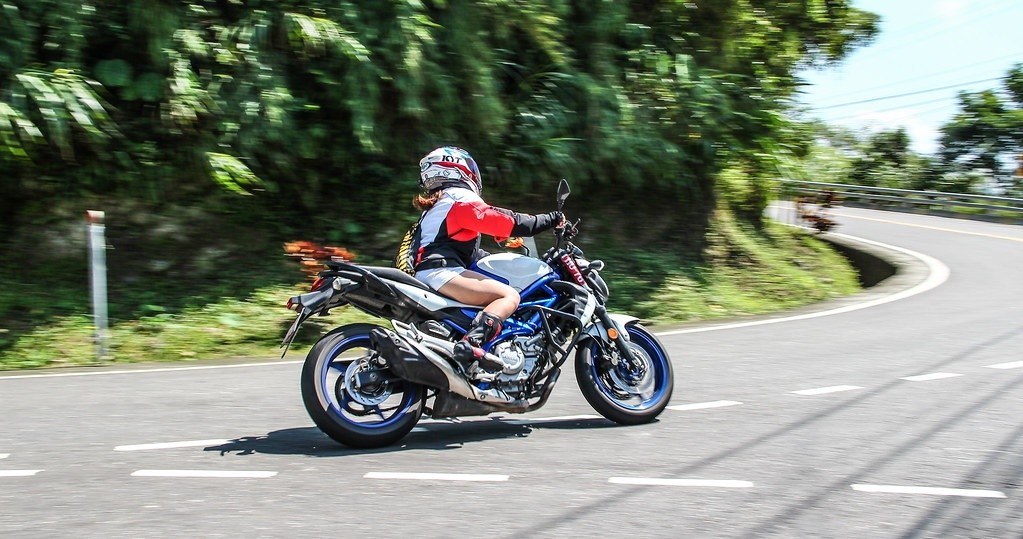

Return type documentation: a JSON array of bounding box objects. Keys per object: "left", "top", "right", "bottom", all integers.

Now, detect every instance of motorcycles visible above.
[{"left": 278, "top": 177, "right": 674, "bottom": 451}]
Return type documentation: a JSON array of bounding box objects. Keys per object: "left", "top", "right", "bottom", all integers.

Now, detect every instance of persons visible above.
[{"left": 414, "top": 146, "right": 566, "bottom": 372}]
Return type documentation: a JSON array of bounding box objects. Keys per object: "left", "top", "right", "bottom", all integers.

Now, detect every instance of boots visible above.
[{"left": 454, "top": 310, "right": 503, "bottom": 372}]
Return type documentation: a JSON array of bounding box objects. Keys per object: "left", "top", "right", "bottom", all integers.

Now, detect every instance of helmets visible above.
[{"left": 420, "top": 146, "right": 481, "bottom": 196}]
